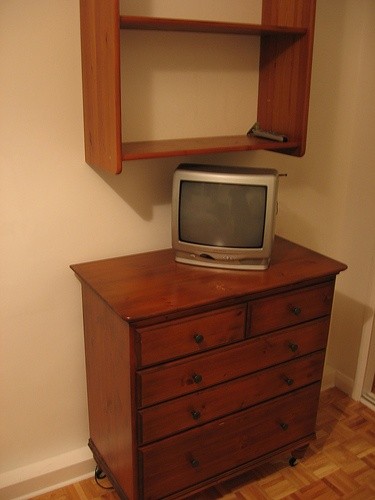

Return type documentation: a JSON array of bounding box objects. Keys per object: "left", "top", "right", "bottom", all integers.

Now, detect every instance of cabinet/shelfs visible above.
[
  {"left": 78, "top": 1, "right": 319, "bottom": 176},
  {"left": 69, "top": 234, "right": 349, "bottom": 500}
]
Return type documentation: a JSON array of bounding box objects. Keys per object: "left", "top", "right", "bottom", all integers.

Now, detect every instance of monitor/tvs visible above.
[{"left": 171, "top": 162, "right": 279, "bottom": 271}]
